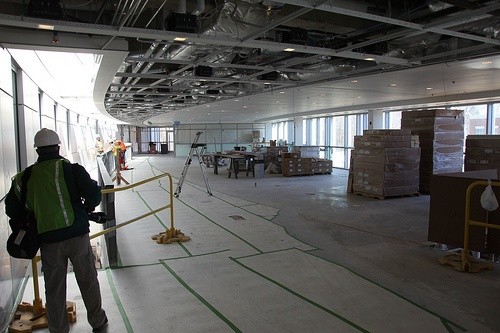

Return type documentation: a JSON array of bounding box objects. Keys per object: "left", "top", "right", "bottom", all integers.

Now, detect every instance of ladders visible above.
[{"left": 173, "top": 132, "right": 213, "bottom": 198}]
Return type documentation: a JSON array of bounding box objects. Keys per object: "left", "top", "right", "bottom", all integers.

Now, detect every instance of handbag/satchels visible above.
[{"left": 6, "top": 212, "right": 39, "bottom": 260}]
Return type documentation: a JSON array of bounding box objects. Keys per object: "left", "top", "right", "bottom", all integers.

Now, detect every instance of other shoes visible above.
[{"left": 92, "top": 316, "right": 108, "bottom": 332}]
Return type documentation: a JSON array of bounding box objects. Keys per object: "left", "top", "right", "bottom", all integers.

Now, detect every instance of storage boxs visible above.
[
  {"left": 352, "top": 108, "right": 500, "bottom": 201},
  {"left": 281, "top": 152, "right": 333, "bottom": 177}
]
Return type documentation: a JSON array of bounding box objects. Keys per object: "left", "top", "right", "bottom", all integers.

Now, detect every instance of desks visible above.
[{"left": 212, "top": 153, "right": 256, "bottom": 179}]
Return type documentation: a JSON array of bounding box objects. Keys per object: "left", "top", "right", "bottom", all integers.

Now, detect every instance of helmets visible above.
[{"left": 33, "top": 128, "right": 62, "bottom": 148}]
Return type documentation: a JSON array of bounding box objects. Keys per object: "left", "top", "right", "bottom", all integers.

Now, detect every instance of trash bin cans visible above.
[
  {"left": 150, "top": 144, "right": 156, "bottom": 154},
  {"left": 161, "top": 144, "right": 168, "bottom": 154}
]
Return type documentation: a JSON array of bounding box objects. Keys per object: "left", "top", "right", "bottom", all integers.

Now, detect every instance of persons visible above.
[
  {"left": 109, "top": 138, "right": 126, "bottom": 170},
  {"left": 5, "top": 128, "right": 108, "bottom": 333}
]
